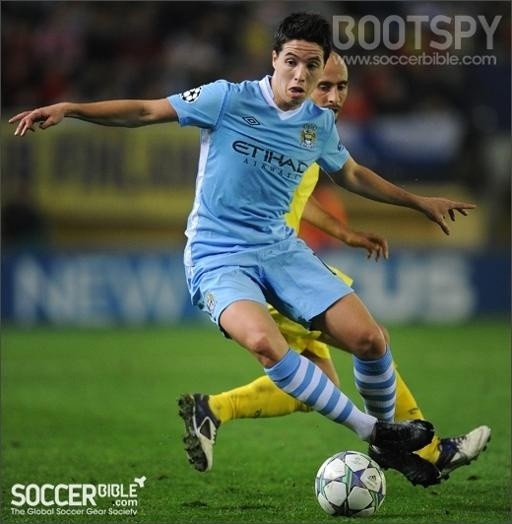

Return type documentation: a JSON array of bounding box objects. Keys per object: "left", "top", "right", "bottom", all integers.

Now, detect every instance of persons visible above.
[
  {"left": 4, "top": 9, "right": 478, "bottom": 489},
  {"left": 172, "top": 47, "right": 493, "bottom": 485}
]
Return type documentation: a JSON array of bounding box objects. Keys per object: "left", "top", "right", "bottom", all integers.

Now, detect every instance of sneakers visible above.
[
  {"left": 369, "top": 419, "right": 491, "bottom": 488},
  {"left": 178, "top": 393, "right": 220, "bottom": 472}
]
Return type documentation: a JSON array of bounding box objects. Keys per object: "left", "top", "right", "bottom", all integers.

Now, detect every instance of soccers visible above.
[{"left": 315, "top": 450, "right": 386, "bottom": 516}]
